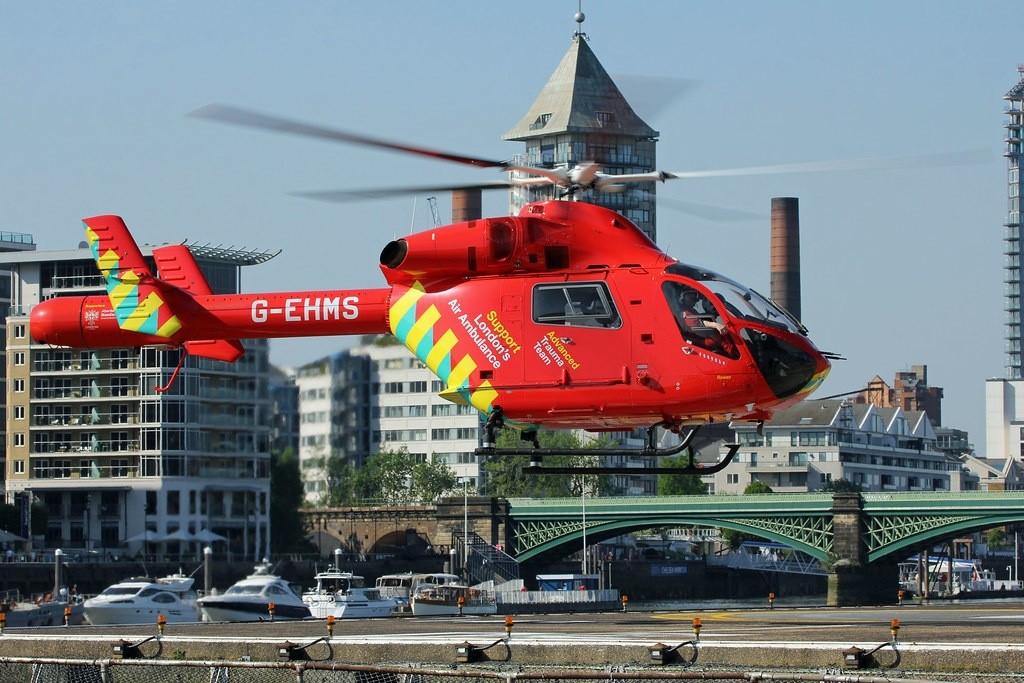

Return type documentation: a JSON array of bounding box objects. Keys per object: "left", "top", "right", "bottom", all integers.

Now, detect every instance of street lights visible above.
[{"left": 1006, "top": 565, "right": 1012, "bottom": 591}]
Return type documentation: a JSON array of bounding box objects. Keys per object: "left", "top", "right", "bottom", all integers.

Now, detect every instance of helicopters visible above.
[{"left": 28, "top": 94, "right": 995, "bottom": 475}]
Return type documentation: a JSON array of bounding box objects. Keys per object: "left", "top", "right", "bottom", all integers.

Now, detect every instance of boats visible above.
[
  {"left": 195, "top": 557, "right": 316, "bottom": 623},
  {"left": 899, "top": 561, "right": 997, "bottom": 597},
  {"left": 375, "top": 571, "right": 498, "bottom": 616},
  {"left": 0, "top": 586, "right": 85, "bottom": 627},
  {"left": 301, "top": 548, "right": 399, "bottom": 618},
  {"left": 83, "top": 568, "right": 202, "bottom": 625}
]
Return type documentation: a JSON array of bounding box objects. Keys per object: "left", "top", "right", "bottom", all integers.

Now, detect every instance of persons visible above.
[{"left": 675, "top": 285, "right": 751, "bottom": 357}]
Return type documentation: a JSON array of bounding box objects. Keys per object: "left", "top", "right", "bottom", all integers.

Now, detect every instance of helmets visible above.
[
  {"left": 702, "top": 293, "right": 727, "bottom": 312},
  {"left": 678, "top": 286, "right": 700, "bottom": 305}
]
